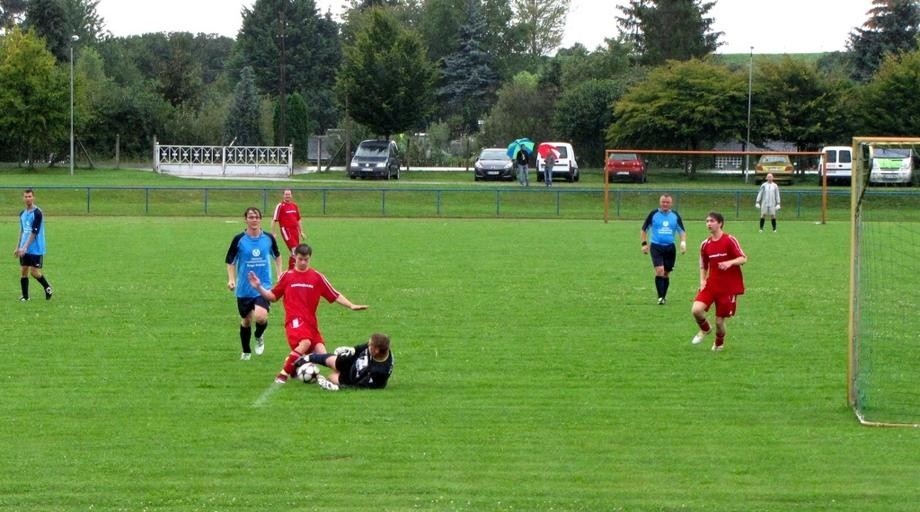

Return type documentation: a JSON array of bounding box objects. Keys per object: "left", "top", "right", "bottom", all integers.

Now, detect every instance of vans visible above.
[
  {"left": 535, "top": 140, "right": 580, "bottom": 183},
  {"left": 348, "top": 139, "right": 402, "bottom": 181},
  {"left": 868, "top": 144, "right": 915, "bottom": 186},
  {"left": 816, "top": 144, "right": 852, "bottom": 186}
]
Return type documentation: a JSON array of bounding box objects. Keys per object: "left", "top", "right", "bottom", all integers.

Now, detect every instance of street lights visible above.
[
  {"left": 742, "top": 44, "right": 757, "bottom": 184},
  {"left": 69, "top": 33, "right": 80, "bottom": 176}
]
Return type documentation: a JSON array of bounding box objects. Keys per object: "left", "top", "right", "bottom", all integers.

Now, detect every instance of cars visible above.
[
  {"left": 601, "top": 152, "right": 649, "bottom": 184},
  {"left": 473, "top": 145, "right": 518, "bottom": 183},
  {"left": 477, "top": 119, "right": 485, "bottom": 127},
  {"left": 753, "top": 154, "right": 798, "bottom": 186}
]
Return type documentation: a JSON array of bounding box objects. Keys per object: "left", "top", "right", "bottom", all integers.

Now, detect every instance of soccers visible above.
[{"left": 297, "top": 362, "right": 320, "bottom": 384}]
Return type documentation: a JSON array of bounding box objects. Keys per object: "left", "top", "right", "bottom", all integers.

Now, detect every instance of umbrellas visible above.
[
  {"left": 506, "top": 137, "right": 535, "bottom": 161},
  {"left": 537, "top": 142, "right": 561, "bottom": 160}
]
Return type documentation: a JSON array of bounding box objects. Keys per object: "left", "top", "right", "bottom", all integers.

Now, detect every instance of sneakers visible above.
[
  {"left": 19, "top": 297, "right": 31, "bottom": 302},
  {"left": 712, "top": 343, "right": 723, "bottom": 352},
  {"left": 276, "top": 374, "right": 288, "bottom": 385},
  {"left": 45, "top": 287, "right": 52, "bottom": 300},
  {"left": 657, "top": 298, "right": 665, "bottom": 304},
  {"left": 240, "top": 353, "right": 251, "bottom": 361},
  {"left": 254, "top": 330, "right": 264, "bottom": 355},
  {"left": 295, "top": 353, "right": 313, "bottom": 367},
  {"left": 692, "top": 325, "right": 712, "bottom": 344}
]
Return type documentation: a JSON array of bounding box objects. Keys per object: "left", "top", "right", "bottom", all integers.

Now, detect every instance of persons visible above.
[
  {"left": 516, "top": 144, "right": 531, "bottom": 187},
  {"left": 14, "top": 190, "right": 52, "bottom": 302},
  {"left": 642, "top": 193, "right": 687, "bottom": 304},
  {"left": 225, "top": 207, "right": 282, "bottom": 360},
  {"left": 691, "top": 212, "right": 748, "bottom": 350},
  {"left": 272, "top": 189, "right": 305, "bottom": 255},
  {"left": 295, "top": 333, "right": 393, "bottom": 390},
  {"left": 544, "top": 149, "right": 555, "bottom": 188},
  {"left": 247, "top": 244, "right": 369, "bottom": 385},
  {"left": 755, "top": 173, "right": 782, "bottom": 233}
]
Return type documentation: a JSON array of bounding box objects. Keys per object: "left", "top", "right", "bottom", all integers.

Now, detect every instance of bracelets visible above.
[
  {"left": 680, "top": 241, "right": 686, "bottom": 245},
  {"left": 641, "top": 241, "right": 647, "bottom": 246}
]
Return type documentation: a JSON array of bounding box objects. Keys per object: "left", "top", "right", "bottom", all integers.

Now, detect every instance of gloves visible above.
[
  {"left": 334, "top": 346, "right": 356, "bottom": 357},
  {"left": 317, "top": 375, "right": 338, "bottom": 391}
]
